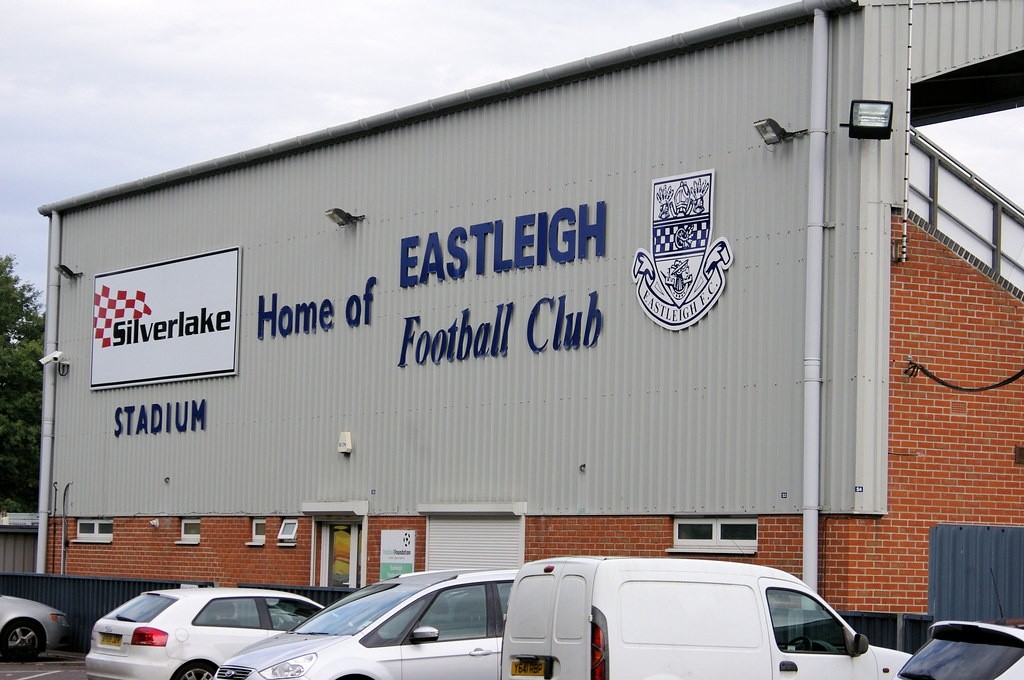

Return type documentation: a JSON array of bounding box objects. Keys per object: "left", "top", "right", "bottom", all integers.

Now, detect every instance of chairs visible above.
[
  {"left": 212, "top": 601, "right": 241, "bottom": 626},
  {"left": 432, "top": 597, "right": 452, "bottom": 630},
  {"left": 454, "top": 593, "right": 481, "bottom": 634}
]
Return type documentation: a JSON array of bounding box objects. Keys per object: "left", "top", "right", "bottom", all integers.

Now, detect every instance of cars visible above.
[
  {"left": 0, "top": 594, "right": 73, "bottom": 663},
  {"left": 214, "top": 568, "right": 519, "bottom": 680},
  {"left": 894, "top": 619, "right": 1024, "bottom": 680},
  {"left": 85, "top": 589, "right": 372, "bottom": 680}
]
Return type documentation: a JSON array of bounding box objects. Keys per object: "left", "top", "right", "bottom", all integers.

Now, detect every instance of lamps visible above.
[
  {"left": 54, "top": 265, "right": 82, "bottom": 280},
  {"left": 325, "top": 208, "right": 365, "bottom": 227},
  {"left": 753, "top": 118, "right": 807, "bottom": 145},
  {"left": 840, "top": 100, "right": 893, "bottom": 140}
]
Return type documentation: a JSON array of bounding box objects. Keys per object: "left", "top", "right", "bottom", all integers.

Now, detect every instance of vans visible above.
[{"left": 499, "top": 557, "right": 913, "bottom": 680}]
